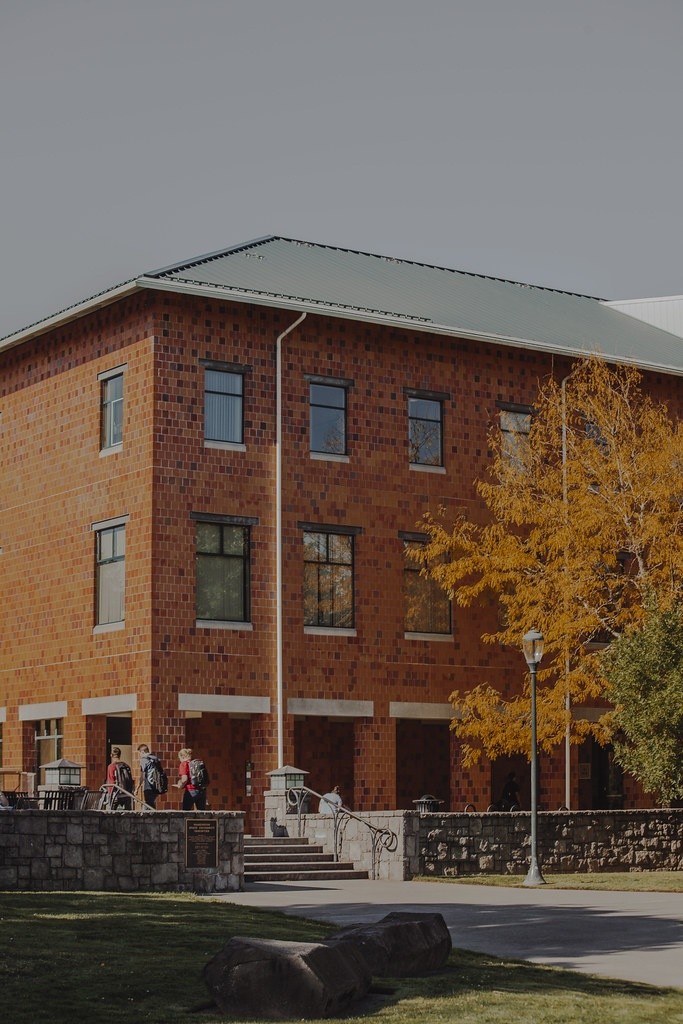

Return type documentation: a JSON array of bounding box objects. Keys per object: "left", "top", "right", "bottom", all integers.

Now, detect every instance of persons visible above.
[
  {"left": 177, "top": 749, "right": 206, "bottom": 810},
  {"left": 500, "top": 773, "right": 522, "bottom": 810},
  {"left": 318, "top": 785, "right": 343, "bottom": 813},
  {"left": 134, "top": 744, "right": 161, "bottom": 810},
  {"left": 108, "top": 747, "right": 131, "bottom": 810}
]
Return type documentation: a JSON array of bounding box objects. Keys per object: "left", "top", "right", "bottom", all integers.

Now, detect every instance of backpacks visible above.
[
  {"left": 112, "top": 762, "right": 133, "bottom": 793},
  {"left": 189, "top": 759, "right": 209, "bottom": 787},
  {"left": 143, "top": 757, "right": 168, "bottom": 794}
]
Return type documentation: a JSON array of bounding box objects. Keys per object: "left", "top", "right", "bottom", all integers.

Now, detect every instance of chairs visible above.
[{"left": 0, "top": 790, "right": 120, "bottom": 811}]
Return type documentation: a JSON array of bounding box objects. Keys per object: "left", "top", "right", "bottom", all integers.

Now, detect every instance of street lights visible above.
[{"left": 522, "top": 628, "right": 546, "bottom": 887}]
